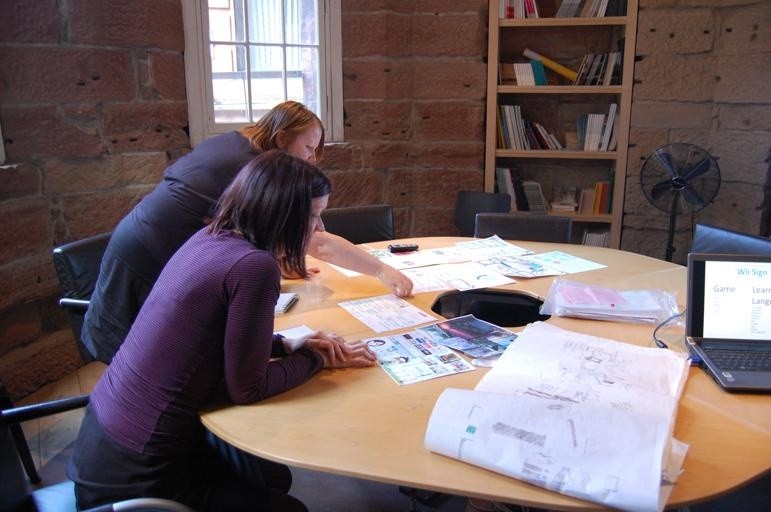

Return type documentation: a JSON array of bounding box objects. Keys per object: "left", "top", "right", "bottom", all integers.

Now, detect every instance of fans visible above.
[{"left": 637, "top": 139, "right": 723, "bottom": 262}]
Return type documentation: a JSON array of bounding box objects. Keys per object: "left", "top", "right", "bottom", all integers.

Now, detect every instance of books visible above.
[
  {"left": 273, "top": 291, "right": 299, "bottom": 314},
  {"left": 497, "top": 1, "right": 627, "bottom": 248}
]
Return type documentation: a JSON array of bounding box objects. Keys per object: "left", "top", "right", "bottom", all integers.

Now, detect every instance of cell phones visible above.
[{"left": 388, "top": 244, "right": 419, "bottom": 253}]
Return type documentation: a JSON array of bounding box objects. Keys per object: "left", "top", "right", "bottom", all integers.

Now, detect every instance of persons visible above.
[
  {"left": 366, "top": 338, "right": 386, "bottom": 351},
  {"left": 78, "top": 100, "right": 414, "bottom": 367},
  {"left": 65, "top": 148, "right": 310, "bottom": 511},
  {"left": 380, "top": 354, "right": 409, "bottom": 367}
]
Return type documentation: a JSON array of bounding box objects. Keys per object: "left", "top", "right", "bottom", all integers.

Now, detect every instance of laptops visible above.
[{"left": 684, "top": 253, "right": 771, "bottom": 395}]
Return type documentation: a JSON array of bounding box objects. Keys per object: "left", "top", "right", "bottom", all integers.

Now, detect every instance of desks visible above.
[{"left": 197, "top": 230, "right": 771, "bottom": 511}]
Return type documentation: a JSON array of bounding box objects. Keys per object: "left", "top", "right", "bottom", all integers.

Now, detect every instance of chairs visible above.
[
  {"left": 474, "top": 212, "right": 571, "bottom": 244},
  {"left": 52, "top": 232, "right": 113, "bottom": 363},
  {"left": 4, "top": 395, "right": 187, "bottom": 510},
  {"left": 691, "top": 224, "right": 770, "bottom": 255},
  {"left": 322, "top": 204, "right": 395, "bottom": 244},
  {"left": 453, "top": 190, "right": 511, "bottom": 237},
  {"left": 2, "top": 386, "right": 43, "bottom": 485}
]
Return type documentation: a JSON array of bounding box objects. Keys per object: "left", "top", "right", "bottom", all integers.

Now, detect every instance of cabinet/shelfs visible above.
[{"left": 484, "top": 0, "right": 638, "bottom": 252}]
